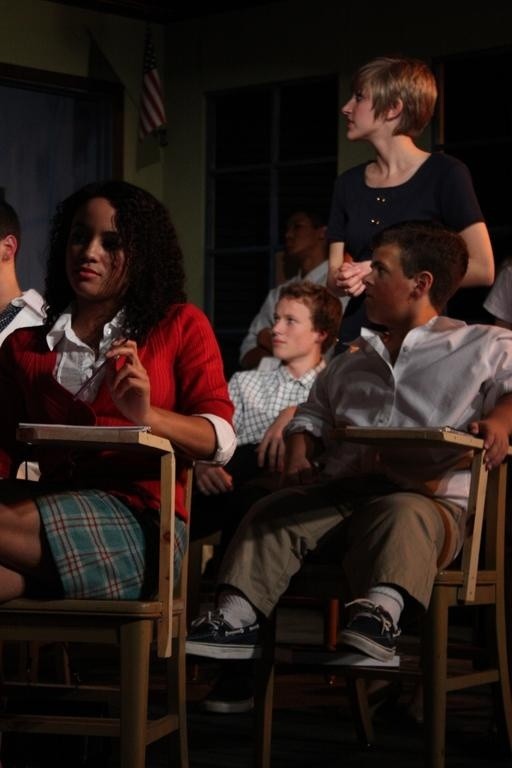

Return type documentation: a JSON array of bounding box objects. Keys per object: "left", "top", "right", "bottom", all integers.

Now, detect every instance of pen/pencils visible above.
[{"left": 72, "top": 330, "right": 138, "bottom": 402}]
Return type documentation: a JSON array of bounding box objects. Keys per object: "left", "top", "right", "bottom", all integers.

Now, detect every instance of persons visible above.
[
  {"left": 184, "top": 225, "right": 512, "bottom": 662},
  {"left": 328, "top": 59, "right": 495, "bottom": 359},
  {"left": 0, "top": 179, "right": 236, "bottom": 607},
  {"left": 484, "top": 258, "right": 512, "bottom": 330},
  {"left": 0, "top": 205, "right": 49, "bottom": 349},
  {"left": 238, "top": 199, "right": 352, "bottom": 371},
  {"left": 190, "top": 282, "right": 342, "bottom": 542}
]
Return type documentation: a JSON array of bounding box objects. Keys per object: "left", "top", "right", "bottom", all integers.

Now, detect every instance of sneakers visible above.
[
  {"left": 201, "top": 674, "right": 278, "bottom": 710},
  {"left": 185, "top": 609, "right": 262, "bottom": 660},
  {"left": 340, "top": 600, "right": 404, "bottom": 664}
]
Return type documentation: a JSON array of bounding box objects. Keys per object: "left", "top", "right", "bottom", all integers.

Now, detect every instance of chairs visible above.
[
  {"left": 0, "top": 458, "right": 191, "bottom": 768},
  {"left": 259, "top": 458, "right": 510, "bottom": 767}
]
